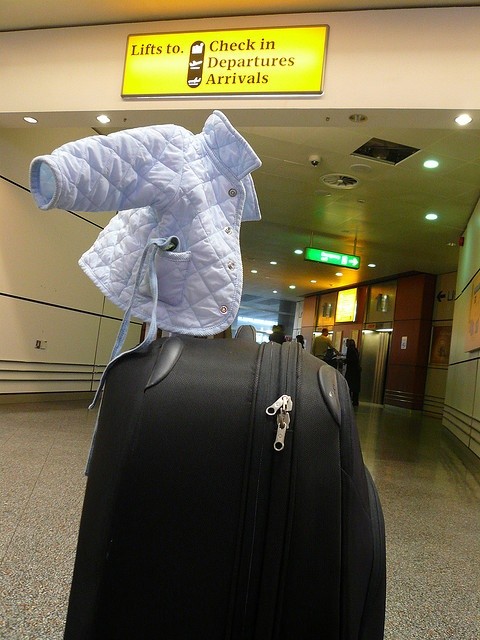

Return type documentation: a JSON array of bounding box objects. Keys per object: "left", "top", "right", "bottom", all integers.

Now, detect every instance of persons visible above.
[
  {"left": 311, "top": 328, "right": 342, "bottom": 361},
  {"left": 269, "top": 324, "right": 287, "bottom": 344},
  {"left": 342, "top": 338, "right": 363, "bottom": 409},
  {"left": 296, "top": 335, "right": 304, "bottom": 349}
]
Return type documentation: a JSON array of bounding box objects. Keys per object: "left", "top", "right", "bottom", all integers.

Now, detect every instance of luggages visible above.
[{"left": 62, "top": 322, "right": 387, "bottom": 639}]
